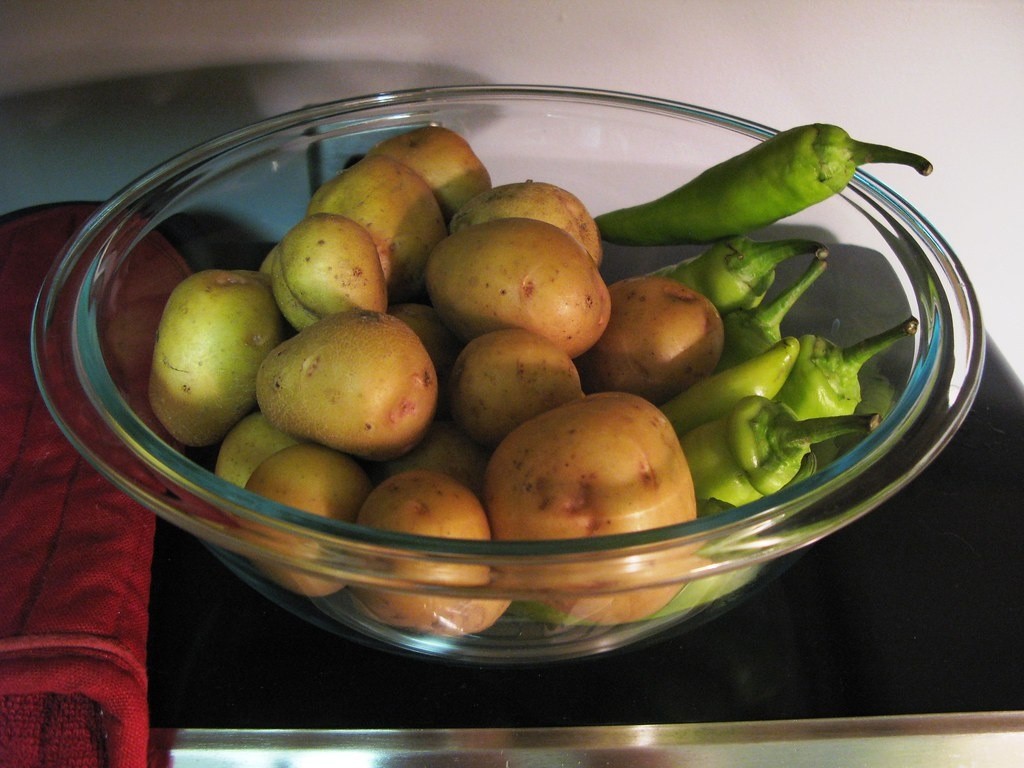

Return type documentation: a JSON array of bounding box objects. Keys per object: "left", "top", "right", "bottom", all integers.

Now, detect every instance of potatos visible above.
[{"left": 148, "top": 124, "right": 721, "bottom": 633}]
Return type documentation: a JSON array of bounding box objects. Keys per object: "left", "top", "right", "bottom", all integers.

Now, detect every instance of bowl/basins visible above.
[{"left": 29, "top": 84, "right": 986, "bottom": 669}]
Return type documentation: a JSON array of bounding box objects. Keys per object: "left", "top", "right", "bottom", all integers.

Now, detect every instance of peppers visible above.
[{"left": 508, "top": 123, "right": 936, "bottom": 625}]
